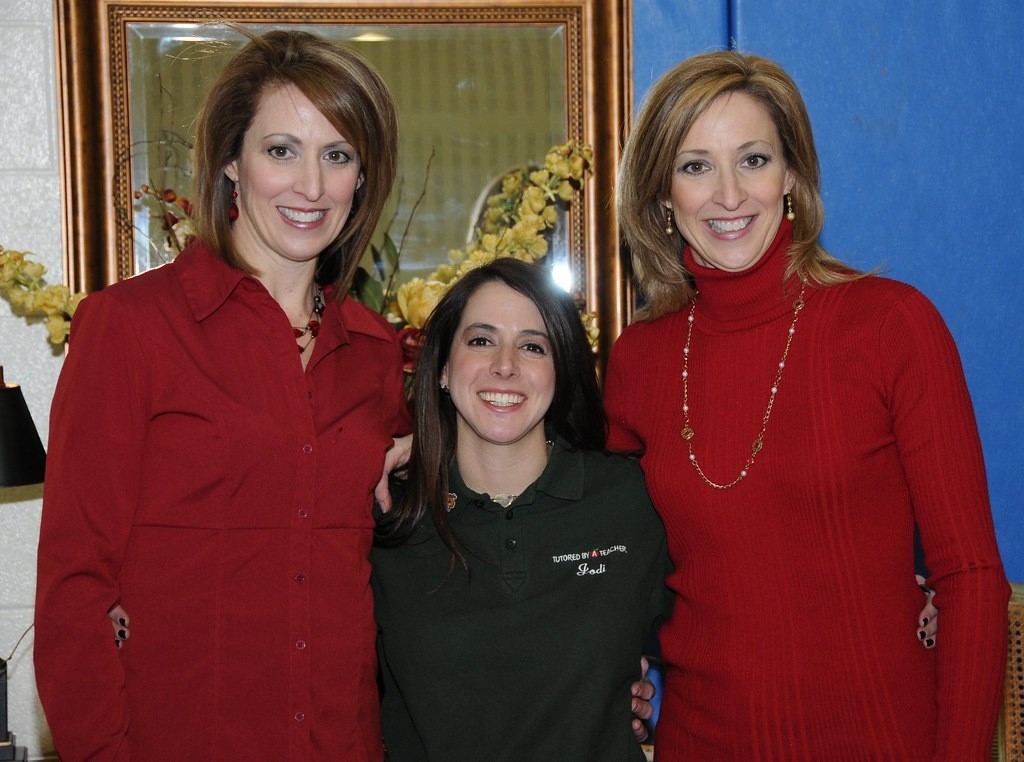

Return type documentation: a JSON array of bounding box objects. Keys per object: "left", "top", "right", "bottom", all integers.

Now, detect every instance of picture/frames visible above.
[{"left": 50, "top": 0, "right": 633, "bottom": 387}]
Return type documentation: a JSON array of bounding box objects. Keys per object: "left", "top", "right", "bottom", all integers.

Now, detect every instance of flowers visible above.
[{"left": 0, "top": 139, "right": 601, "bottom": 376}]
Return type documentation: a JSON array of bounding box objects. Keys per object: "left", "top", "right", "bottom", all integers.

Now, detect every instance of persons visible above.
[
  {"left": 30, "top": 28, "right": 656, "bottom": 762},
  {"left": 108, "top": 259, "right": 944, "bottom": 762},
  {"left": 375, "top": 49, "right": 1006, "bottom": 761}
]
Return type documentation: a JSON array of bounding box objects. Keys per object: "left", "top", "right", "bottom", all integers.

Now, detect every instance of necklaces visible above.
[
  {"left": 684, "top": 270, "right": 815, "bottom": 489},
  {"left": 492, "top": 437, "right": 556, "bottom": 506},
  {"left": 293, "top": 283, "right": 324, "bottom": 353}
]
